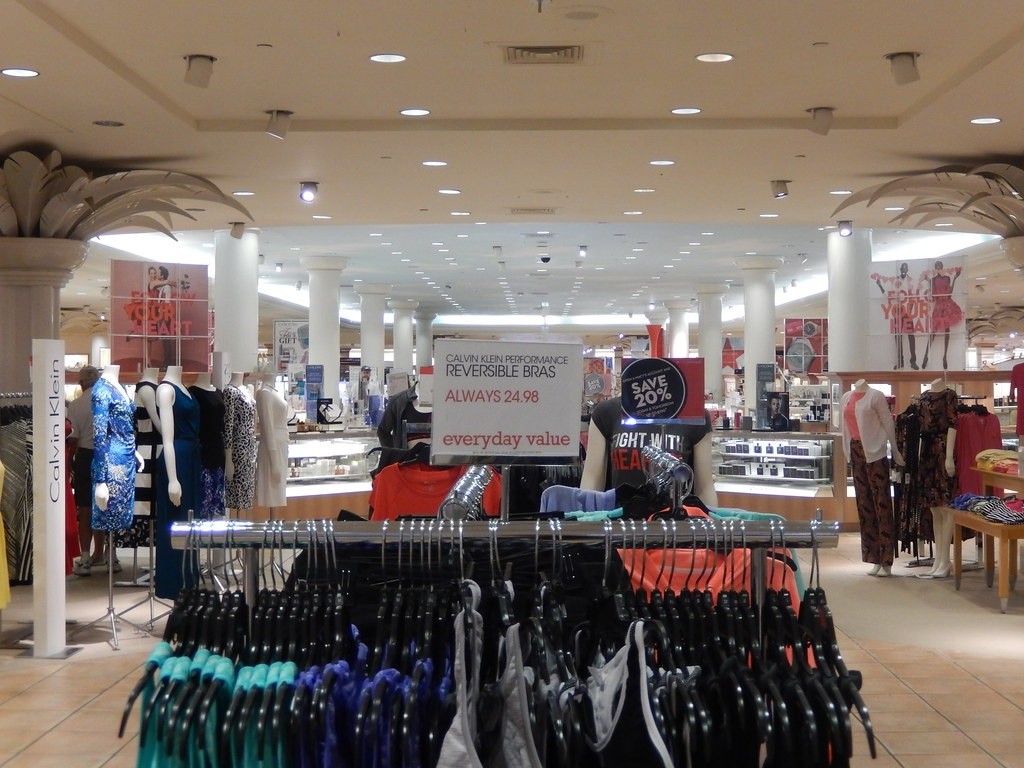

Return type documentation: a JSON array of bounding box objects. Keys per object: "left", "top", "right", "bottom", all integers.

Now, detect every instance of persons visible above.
[
  {"left": 769, "top": 396, "right": 788, "bottom": 430},
  {"left": 378, "top": 382, "right": 432, "bottom": 449},
  {"left": 918, "top": 378, "right": 958, "bottom": 578},
  {"left": 580, "top": 398, "right": 718, "bottom": 507},
  {"left": 91, "top": 366, "right": 257, "bottom": 600},
  {"left": 257, "top": 373, "right": 290, "bottom": 505},
  {"left": 126, "top": 265, "right": 177, "bottom": 371},
  {"left": 351, "top": 366, "right": 379, "bottom": 396},
  {"left": 840, "top": 379, "right": 906, "bottom": 576},
  {"left": 64, "top": 367, "right": 122, "bottom": 574},
  {"left": 876, "top": 261, "right": 961, "bottom": 369},
  {"left": 1010, "top": 363, "right": 1024, "bottom": 446}
]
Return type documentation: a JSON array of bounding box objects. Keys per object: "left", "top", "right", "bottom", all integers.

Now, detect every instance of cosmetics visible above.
[{"left": 719, "top": 443, "right": 822, "bottom": 479}]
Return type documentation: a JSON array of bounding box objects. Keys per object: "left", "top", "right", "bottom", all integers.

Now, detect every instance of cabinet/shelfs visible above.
[
  {"left": 230, "top": 428, "right": 381, "bottom": 522},
  {"left": 709, "top": 428, "right": 894, "bottom": 532}
]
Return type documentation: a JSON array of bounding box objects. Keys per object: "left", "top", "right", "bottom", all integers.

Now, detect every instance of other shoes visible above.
[{"left": 88, "top": 554, "right": 107, "bottom": 566}]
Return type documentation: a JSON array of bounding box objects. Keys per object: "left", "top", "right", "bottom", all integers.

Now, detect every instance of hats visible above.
[{"left": 361, "top": 366, "right": 371, "bottom": 371}]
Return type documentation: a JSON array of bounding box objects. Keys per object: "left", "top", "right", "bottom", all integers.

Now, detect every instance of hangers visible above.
[
  {"left": 899, "top": 394, "right": 989, "bottom": 421},
  {"left": 120, "top": 446, "right": 877, "bottom": 768},
  {"left": 0, "top": 392, "right": 71, "bottom": 426}
]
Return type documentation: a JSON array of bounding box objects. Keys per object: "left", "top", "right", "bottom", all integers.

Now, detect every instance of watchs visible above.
[{"left": 786, "top": 323, "right": 828, "bottom": 372}]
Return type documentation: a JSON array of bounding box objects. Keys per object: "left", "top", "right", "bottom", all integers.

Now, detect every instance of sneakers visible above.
[
  {"left": 72, "top": 560, "right": 91, "bottom": 576},
  {"left": 106, "top": 558, "right": 122, "bottom": 573}
]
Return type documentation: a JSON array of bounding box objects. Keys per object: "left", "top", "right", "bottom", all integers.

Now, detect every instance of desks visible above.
[
  {"left": 942, "top": 507, "right": 1024, "bottom": 614},
  {"left": 971, "top": 466, "right": 1024, "bottom": 569}
]
